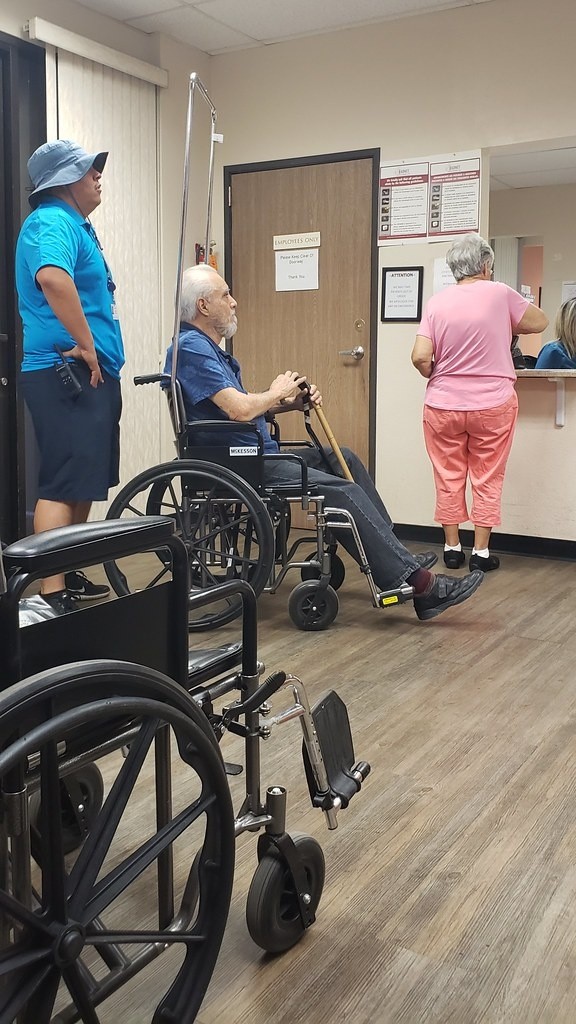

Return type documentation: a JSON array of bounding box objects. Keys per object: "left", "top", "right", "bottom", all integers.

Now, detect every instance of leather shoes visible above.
[
  {"left": 413, "top": 569, "right": 484, "bottom": 620},
  {"left": 415, "top": 552, "right": 438, "bottom": 570}
]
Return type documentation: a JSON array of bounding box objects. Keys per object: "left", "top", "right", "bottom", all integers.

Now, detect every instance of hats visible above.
[{"left": 27, "top": 140, "right": 109, "bottom": 210}]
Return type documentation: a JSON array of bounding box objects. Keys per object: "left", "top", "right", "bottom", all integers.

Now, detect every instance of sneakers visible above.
[
  {"left": 64, "top": 570, "right": 111, "bottom": 601},
  {"left": 39, "top": 590, "right": 80, "bottom": 615}
]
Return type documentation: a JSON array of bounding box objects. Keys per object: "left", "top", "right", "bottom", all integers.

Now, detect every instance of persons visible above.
[
  {"left": 412, "top": 230, "right": 549, "bottom": 573},
  {"left": 535, "top": 297, "right": 576, "bottom": 369},
  {"left": 164, "top": 264, "right": 484, "bottom": 623},
  {"left": 16, "top": 138, "right": 126, "bottom": 619}
]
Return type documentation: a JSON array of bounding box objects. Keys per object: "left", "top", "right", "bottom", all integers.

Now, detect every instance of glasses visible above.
[{"left": 489, "top": 268, "right": 494, "bottom": 276}]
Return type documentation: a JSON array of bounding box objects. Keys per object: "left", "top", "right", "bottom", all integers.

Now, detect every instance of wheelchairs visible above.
[
  {"left": 0, "top": 517, "right": 371, "bottom": 1024},
  {"left": 103, "top": 373, "right": 416, "bottom": 632}
]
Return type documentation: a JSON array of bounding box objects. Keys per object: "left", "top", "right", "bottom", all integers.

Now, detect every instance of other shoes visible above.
[
  {"left": 443, "top": 550, "right": 466, "bottom": 569},
  {"left": 469, "top": 554, "right": 499, "bottom": 572}
]
